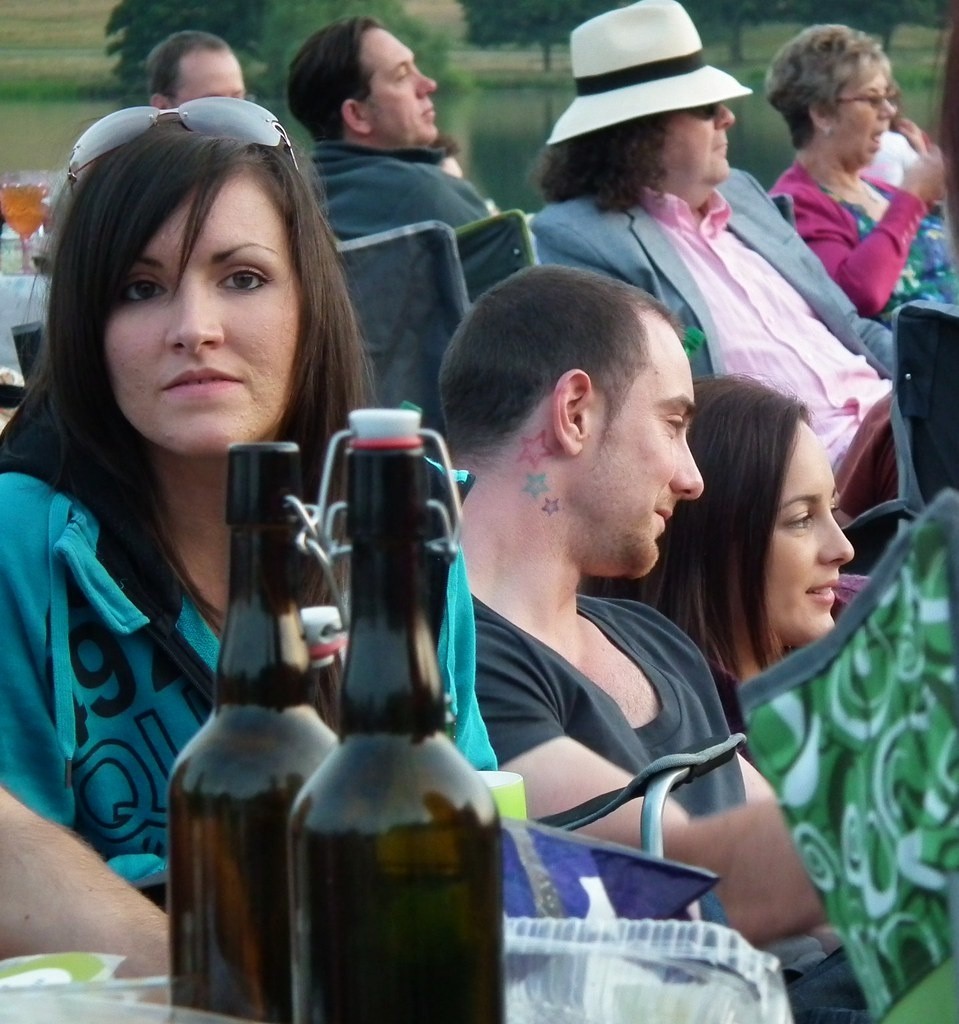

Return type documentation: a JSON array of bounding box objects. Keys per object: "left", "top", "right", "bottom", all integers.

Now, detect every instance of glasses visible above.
[
  {"left": 66, "top": 95, "right": 300, "bottom": 212},
  {"left": 833, "top": 87, "right": 903, "bottom": 109}
]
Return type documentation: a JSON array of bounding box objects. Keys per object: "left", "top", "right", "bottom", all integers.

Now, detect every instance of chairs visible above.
[{"left": 4, "top": 204, "right": 959, "bottom": 927}]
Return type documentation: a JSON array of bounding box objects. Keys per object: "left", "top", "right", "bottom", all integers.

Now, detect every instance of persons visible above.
[
  {"left": 139, "top": 29, "right": 246, "bottom": 113},
  {"left": 282, "top": 15, "right": 493, "bottom": 243},
  {"left": 426, "top": 134, "right": 501, "bottom": 223},
  {"left": 0, "top": 92, "right": 499, "bottom": 1024},
  {"left": 581, "top": 372, "right": 854, "bottom": 688},
  {"left": 862, "top": 90, "right": 927, "bottom": 192},
  {"left": 756, "top": 24, "right": 945, "bottom": 319},
  {"left": 420, "top": 262, "right": 878, "bottom": 1022},
  {"left": 525, "top": 0, "right": 898, "bottom": 518}
]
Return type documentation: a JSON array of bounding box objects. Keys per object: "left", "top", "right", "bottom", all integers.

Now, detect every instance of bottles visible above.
[
  {"left": 282, "top": 403, "right": 510, "bottom": 1024},
  {"left": 164, "top": 439, "right": 354, "bottom": 1024}
]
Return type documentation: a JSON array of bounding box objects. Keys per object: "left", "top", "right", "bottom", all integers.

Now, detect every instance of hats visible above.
[{"left": 544, "top": 0, "right": 756, "bottom": 144}]
